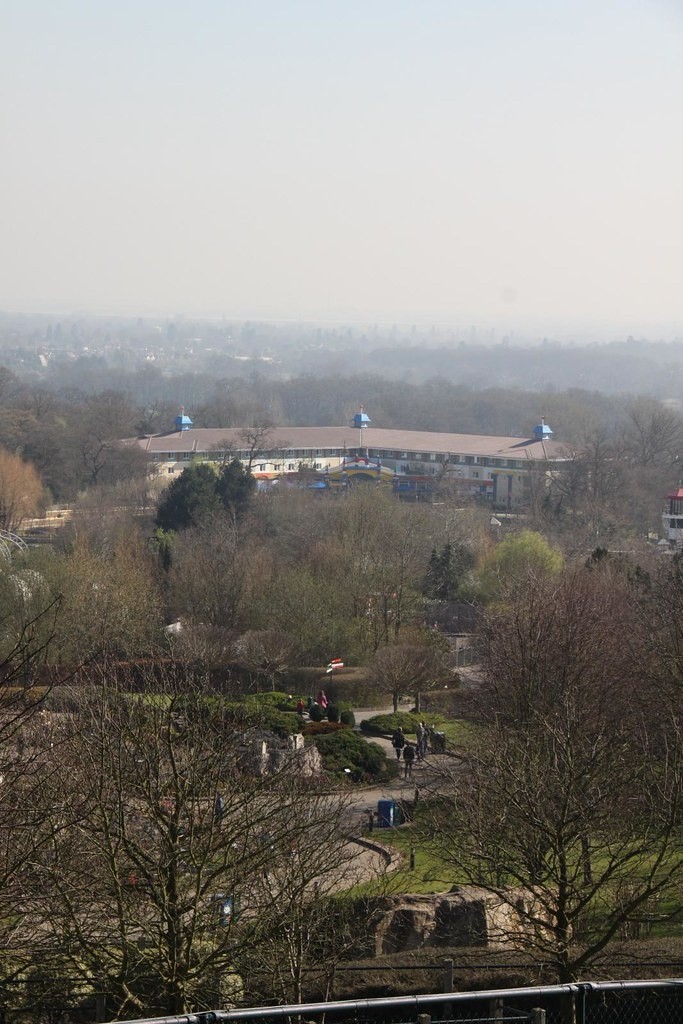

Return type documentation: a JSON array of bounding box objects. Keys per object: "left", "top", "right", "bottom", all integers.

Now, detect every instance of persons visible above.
[
  {"left": 392, "top": 727, "right": 405, "bottom": 762},
  {"left": 297, "top": 698, "right": 304, "bottom": 718},
  {"left": 214, "top": 791, "right": 224, "bottom": 826},
  {"left": 416, "top": 721, "right": 435, "bottom": 756},
  {"left": 317, "top": 690, "right": 328, "bottom": 720},
  {"left": 403, "top": 742, "right": 415, "bottom": 778}
]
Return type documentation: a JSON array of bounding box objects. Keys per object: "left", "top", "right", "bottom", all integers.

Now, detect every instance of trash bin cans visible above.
[
  {"left": 431, "top": 733, "right": 445, "bottom": 754},
  {"left": 378, "top": 799, "right": 395, "bottom": 827},
  {"left": 211, "top": 894, "right": 233, "bottom": 927}
]
